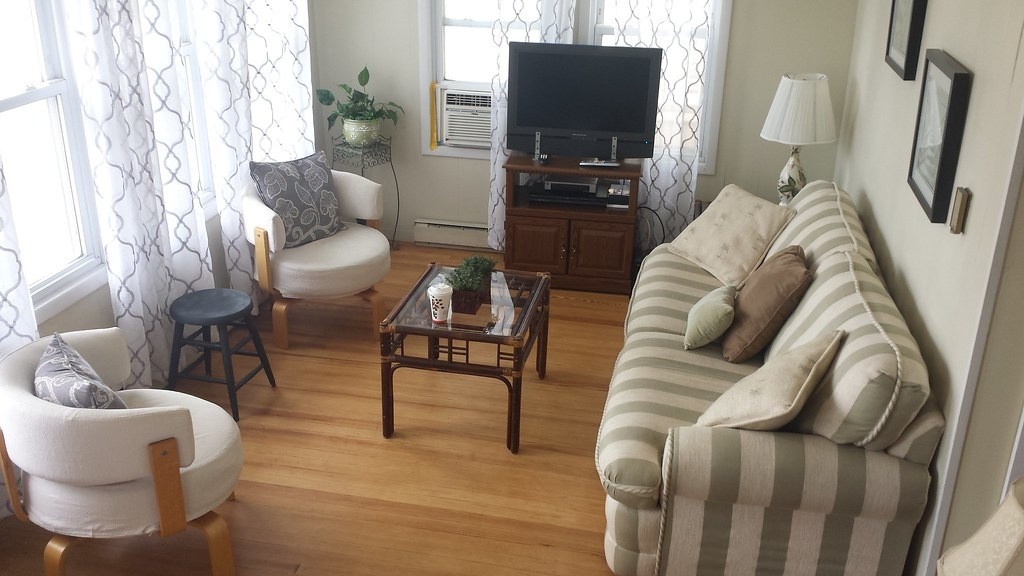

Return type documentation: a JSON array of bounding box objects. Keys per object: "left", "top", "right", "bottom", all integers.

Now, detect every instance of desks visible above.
[
  {"left": 379, "top": 260, "right": 551, "bottom": 455},
  {"left": 331, "top": 132, "right": 400, "bottom": 249}
]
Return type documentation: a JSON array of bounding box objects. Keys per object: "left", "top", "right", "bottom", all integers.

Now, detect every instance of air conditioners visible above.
[{"left": 442, "top": 90, "right": 493, "bottom": 149}]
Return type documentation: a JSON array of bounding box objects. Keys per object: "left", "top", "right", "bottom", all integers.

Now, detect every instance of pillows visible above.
[
  {"left": 34, "top": 331, "right": 127, "bottom": 410},
  {"left": 684, "top": 284, "right": 735, "bottom": 350},
  {"left": 667, "top": 184, "right": 798, "bottom": 291},
  {"left": 695, "top": 328, "right": 847, "bottom": 428},
  {"left": 248, "top": 148, "right": 345, "bottom": 251},
  {"left": 722, "top": 243, "right": 816, "bottom": 363}
]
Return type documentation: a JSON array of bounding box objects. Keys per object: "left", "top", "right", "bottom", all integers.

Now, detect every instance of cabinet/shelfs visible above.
[{"left": 502, "top": 151, "right": 643, "bottom": 293}]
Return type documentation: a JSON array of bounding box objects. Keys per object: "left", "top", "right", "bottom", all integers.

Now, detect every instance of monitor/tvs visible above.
[{"left": 505, "top": 42, "right": 662, "bottom": 160}]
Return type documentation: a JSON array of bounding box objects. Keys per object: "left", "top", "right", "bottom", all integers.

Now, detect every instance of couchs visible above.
[{"left": 594, "top": 178, "right": 947, "bottom": 576}]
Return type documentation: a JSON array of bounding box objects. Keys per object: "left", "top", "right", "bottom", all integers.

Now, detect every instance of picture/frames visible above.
[
  {"left": 906, "top": 47, "right": 971, "bottom": 223},
  {"left": 884, "top": 0, "right": 928, "bottom": 81}
]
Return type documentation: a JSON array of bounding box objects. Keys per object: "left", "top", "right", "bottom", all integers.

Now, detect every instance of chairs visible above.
[
  {"left": 0, "top": 327, "right": 240, "bottom": 576},
  {"left": 244, "top": 169, "right": 392, "bottom": 351}
]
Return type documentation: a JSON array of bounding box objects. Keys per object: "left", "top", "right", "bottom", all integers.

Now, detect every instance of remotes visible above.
[{"left": 579, "top": 159, "right": 620, "bottom": 169}]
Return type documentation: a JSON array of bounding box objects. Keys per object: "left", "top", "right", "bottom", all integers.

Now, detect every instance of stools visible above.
[{"left": 167, "top": 288, "right": 276, "bottom": 423}]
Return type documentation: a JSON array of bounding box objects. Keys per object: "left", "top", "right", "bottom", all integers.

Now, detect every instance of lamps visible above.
[
  {"left": 760, "top": 72, "right": 837, "bottom": 203},
  {"left": 936, "top": 474, "right": 1024, "bottom": 576}
]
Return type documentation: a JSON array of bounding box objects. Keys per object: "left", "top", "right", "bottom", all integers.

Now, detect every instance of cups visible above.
[{"left": 428, "top": 283, "right": 453, "bottom": 324}]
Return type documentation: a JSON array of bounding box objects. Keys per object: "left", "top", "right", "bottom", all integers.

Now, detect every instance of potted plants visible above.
[
  {"left": 445, "top": 255, "right": 497, "bottom": 314},
  {"left": 314, "top": 66, "right": 405, "bottom": 147}
]
